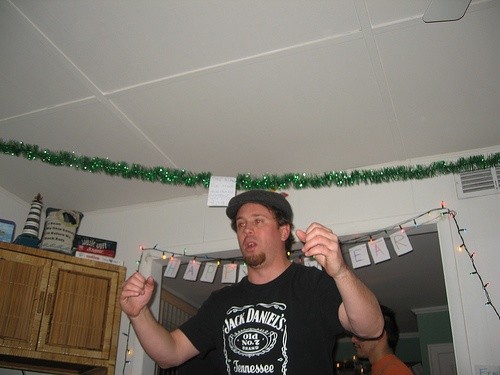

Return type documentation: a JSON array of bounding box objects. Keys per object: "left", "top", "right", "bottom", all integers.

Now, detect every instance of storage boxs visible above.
[
  {"left": 74, "top": 251, "right": 125, "bottom": 267},
  {"left": 77, "top": 245, "right": 115, "bottom": 258},
  {"left": 0, "top": 218, "right": 17, "bottom": 243},
  {"left": 72, "top": 234, "right": 118, "bottom": 258}
]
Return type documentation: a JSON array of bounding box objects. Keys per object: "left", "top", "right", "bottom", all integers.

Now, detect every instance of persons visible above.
[
  {"left": 352, "top": 304, "right": 416, "bottom": 375},
  {"left": 119, "top": 190, "right": 384, "bottom": 375}
]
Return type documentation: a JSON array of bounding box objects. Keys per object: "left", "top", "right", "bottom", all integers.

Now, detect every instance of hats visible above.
[{"left": 225, "top": 190, "right": 294, "bottom": 222}]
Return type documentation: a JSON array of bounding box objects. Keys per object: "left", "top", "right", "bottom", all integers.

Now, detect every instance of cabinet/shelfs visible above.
[{"left": 0, "top": 240, "right": 127, "bottom": 375}]
